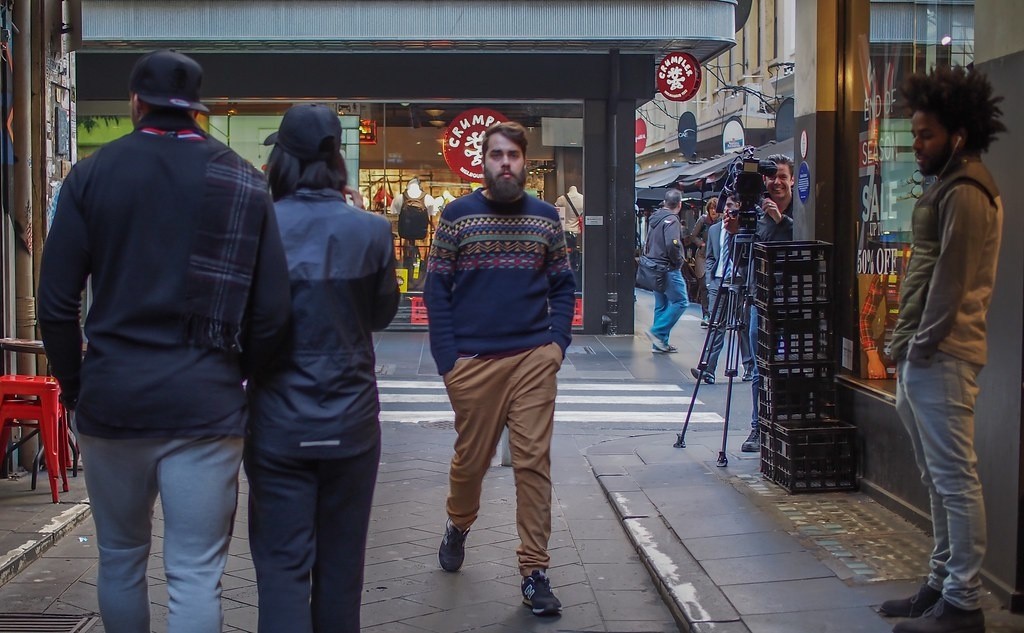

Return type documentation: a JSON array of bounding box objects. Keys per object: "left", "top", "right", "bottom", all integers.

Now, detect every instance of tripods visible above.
[{"left": 673, "top": 211, "right": 766, "bottom": 469}]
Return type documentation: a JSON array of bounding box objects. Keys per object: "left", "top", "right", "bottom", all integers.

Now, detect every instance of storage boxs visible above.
[
  {"left": 757, "top": 366, "right": 837, "bottom": 421},
  {"left": 747, "top": 238, "right": 836, "bottom": 309},
  {"left": 772, "top": 420, "right": 860, "bottom": 496},
  {"left": 396, "top": 268, "right": 408, "bottom": 292},
  {"left": 410, "top": 296, "right": 429, "bottom": 324},
  {"left": 754, "top": 303, "right": 834, "bottom": 366},
  {"left": 572, "top": 298, "right": 582, "bottom": 327},
  {"left": 753, "top": 415, "right": 776, "bottom": 483}
]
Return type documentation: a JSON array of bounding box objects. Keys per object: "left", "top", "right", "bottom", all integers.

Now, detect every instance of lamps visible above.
[
  {"left": 535, "top": 171, "right": 539, "bottom": 175},
  {"left": 536, "top": 161, "right": 555, "bottom": 172},
  {"left": 422, "top": 109, "right": 446, "bottom": 117},
  {"left": 528, "top": 169, "right": 534, "bottom": 175},
  {"left": 429, "top": 120, "right": 447, "bottom": 128}
]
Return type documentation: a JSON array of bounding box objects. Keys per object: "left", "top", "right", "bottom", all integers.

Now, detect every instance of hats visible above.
[
  {"left": 263, "top": 104, "right": 342, "bottom": 160},
  {"left": 128, "top": 48, "right": 210, "bottom": 112}
]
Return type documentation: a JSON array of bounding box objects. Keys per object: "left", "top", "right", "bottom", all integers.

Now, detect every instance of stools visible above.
[{"left": 0, "top": 369, "right": 72, "bottom": 504}]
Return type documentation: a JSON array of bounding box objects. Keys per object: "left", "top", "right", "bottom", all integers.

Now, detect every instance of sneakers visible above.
[
  {"left": 437, "top": 518, "right": 470, "bottom": 572},
  {"left": 881, "top": 576, "right": 942, "bottom": 616},
  {"left": 741, "top": 429, "right": 760, "bottom": 451},
  {"left": 521, "top": 570, "right": 562, "bottom": 613},
  {"left": 891, "top": 599, "right": 986, "bottom": 633}
]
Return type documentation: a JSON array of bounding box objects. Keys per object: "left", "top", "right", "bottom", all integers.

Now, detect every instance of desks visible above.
[{"left": 0, "top": 335, "right": 90, "bottom": 491}]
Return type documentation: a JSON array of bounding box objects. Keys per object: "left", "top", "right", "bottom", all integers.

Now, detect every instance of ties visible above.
[{"left": 722, "top": 231, "right": 731, "bottom": 280}]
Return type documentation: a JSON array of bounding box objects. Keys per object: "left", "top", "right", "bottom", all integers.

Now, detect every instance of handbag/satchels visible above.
[
  {"left": 635, "top": 254, "right": 658, "bottom": 292},
  {"left": 579, "top": 217, "right": 582, "bottom": 232}
]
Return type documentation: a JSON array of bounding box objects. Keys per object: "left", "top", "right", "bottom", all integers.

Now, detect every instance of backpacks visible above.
[{"left": 396, "top": 190, "right": 428, "bottom": 239}]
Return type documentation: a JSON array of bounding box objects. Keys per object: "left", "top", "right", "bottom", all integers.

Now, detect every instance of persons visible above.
[
  {"left": 244, "top": 104, "right": 401, "bottom": 632},
  {"left": 876, "top": 61, "right": 1004, "bottom": 633},
  {"left": 390, "top": 178, "right": 436, "bottom": 216},
  {"left": 690, "top": 198, "right": 723, "bottom": 327},
  {"left": 691, "top": 193, "right": 754, "bottom": 384},
  {"left": 724, "top": 154, "right": 795, "bottom": 453},
  {"left": 641, "top": 190, "right": 688, "bottom": 353},
  {"left": 555, "top": 186, "right": 583, "bottom": 291},
  {"left": 423, "top": 122, "right": 577, "bottom": 614},
  {"left": 36, "top": 47, "right": 289, "bottom": 632},
  {"left": 434, "top": 191, "right": 457, "bottom": 215}
]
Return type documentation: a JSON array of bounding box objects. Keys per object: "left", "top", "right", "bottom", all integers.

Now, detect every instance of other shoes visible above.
[
  {"left": 701, "top": 316, "right": 709, "bottom": 329},
  {"left": 652, "top": 345, "right": 678, "bottom": 352},
  {"left": 646, "top": 331, "right": 667, "bottom": 351},
  {"left": 691, "top": 368, "right": 715, "bottom": 384},
  {"left": 742, "top": 370, "right": 754, "bottom": 381}
]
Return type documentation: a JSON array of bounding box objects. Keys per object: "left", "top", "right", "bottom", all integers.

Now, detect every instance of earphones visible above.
[{"left": 954, "top": 136, "right": 963, "bottom": 147}]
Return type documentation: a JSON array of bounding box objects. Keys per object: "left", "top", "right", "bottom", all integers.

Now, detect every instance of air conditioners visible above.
[{"left": 742, "top": 82, "right": 760, "bottom": 111}]
